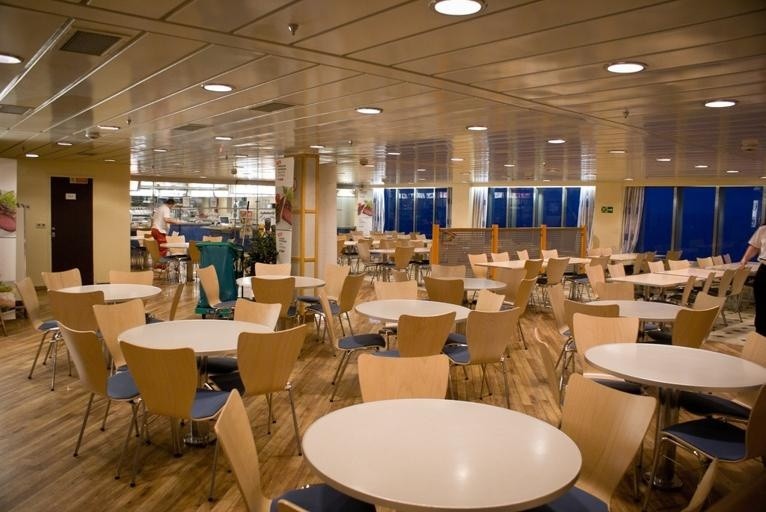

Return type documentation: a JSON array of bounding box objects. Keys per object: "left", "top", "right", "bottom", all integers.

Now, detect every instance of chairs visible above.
[
  {"left": 355, "top": 237, "right": 374, "bottom": 275},
  {"left": 663, "top": 250, "right": 684, "bottom": 269},
  {"left": 599, "top": 246, "right": 610, "bottom": 254},
  {"left": 646, "top": 260, "right": 666, "bottom": 273},
  {"left": 375, "top": 238, "right": 396, "bottom": 249},
  {"left": 372, "top": 312, "right": 457, "bottom": 395},
  {"left": 594, "top": 280, "right": 635, "bottom": 300},
  {"left": 541, "top": 250, "right": 561, "bottom": 258},
  {"left": 588, "top": 256, "right": 611, "bottom": 273},
  {"left": 142, "top": 235, "right": 173, "bottom": 279},
  {"left": 108, "top": 271, "right": 153, "bottom": 350},
  {"left": 407, "top": 240, "right": 426, "bottom": 248},
  {"left": 447, "top": 288, "right": 507, "bottom": 382},
  {"left": 501, "top": 277, "right": 539, "bottom": 351},
  {"left": 138, "top": 229, "right": 154, "bottom": 249},
  {"left": 41, "top": 268, "right": 82, "bottom": 372},
  {"left": 490, "top": 250, "right": 509, "bottom": 261},
  {"left": 583, "top": 264, "right": 607, "bottom": 299},
  {"left": 214, "top": 386, "right": 376, "bottom": 512},
  {"left": 712, "top": 268, "right": 737, "bottom": 332},
  {"left": 146, "top": 284, "right": 184, "bottom": 321},
  {"left": 668, "top": 259, "right": 691, "bottom": 269},
  {"left": 475, "top": 258, "right": 594, "bottom": 278},
  {"left": 547, "top": 282, "right": 569, "bottom": 376},
  {"left": 194, "top": 266, "right": 240, "bottom": 320},
  {"left": 643, "top": 250, "right": 658, "bottom": 271},
  {"left": 318, "top": 290, "right": 387, "bottom": 403},
  {"left": 166, "top": 235, "right": 188, "bottom": 277},
  {"left": 202, "top": 235, "right": 222, "bottom": 241},
  {"left": 712, "top": 255, "right": 724, "bottom": 265},
  {"left": 233, "top": 321, "right": 307, "bottom": 457},
  {"left": 13, "top": 276, "right": 71, "bottom": 391},
  {"left": 55, "top": 319, "right": 151, "bottom": 483},
  {"left": 561, "top": 297, "right": 620, "bottom": 361},
  {"left": 391, "top": 247, "right": 414, "bottom": 274},
  {"left": 641, "top": 385, "right": 766, "bottom": 512},
  {"left": 605, "top": 262, "right": 627, "bottom": 279},
  {"left": 350, "top": 231, "right": 426, "bottom": 241},
  {"left": 231, "top": 296, "right": 282, "bottom": 426},
  {"left": 119, "top": 340, "right": 231, "bottom": 488},
  {"left": 516, "top": 249, "right": 530, "bottom": 262},
  {"left": 701, "top": 270, "right": 716, "bottom": 296},
  {"left": 91, "top": 299, "right": 149, "bottom": 435},
  {"left": 696, "top": 256, "right": 713, "bottom": 267},
  {"left": 572, "top": 313, "right": 639, "bottom": 379},
  {"left": 468, "top": 253, "right": 490, "bottom": 278},
  {"left": 521, "top": 260, "right": 543, "bottom": 310},
  {"left": 672, "top": 305, "right": 720, "bottom": 348},
  {"left": 721, "top": 252, "right": 733, "bottom": 263},
  {"left": 296, "top": 265, "right": 350, "bottom": 336},
  {"left": 536, "top": 370, "right": 657, "bottom": 511},
  {"left": 442, "top": 305, "right": 521, "bottom": 405},
  {"left": 371, "top": 280, "right": 418, "bottom": 299},
  {"left": 428, "top": 263, "right": 467, "bottom": 279},
  {"left": 500, "top": 268, "right": 524, "bottom": 303},
  {"left": 252, "top": 261, "right": 291, "bottom": 277},
  {"left": 719, "top": 268, "right": 751, "bottom": 326},
  {"left": 543, "top": 257, "right": 569, "bottom": 310},
  {"left": 629, "top": 251, "right": 644, "bottom": 274},
  {"left": 659, "top": 330, "right": 766, "bottom": 425},
  {"left": 303, "top": 274, "right": 367, "bottom": 345},
  {"left": 250, "top": 278, "right": 296, "bottom": 321},
  {"left": 673, "top": 274, "right": 696, "bottom": 306},
  {"left": 422, "top": 277, "right": 465, "bottom": 304},
  {"left": 584, "top": 247, "right": 603, "bottom": 258},
  {"left": 376, "top": 246, "right": 414, "bottom": 282}
]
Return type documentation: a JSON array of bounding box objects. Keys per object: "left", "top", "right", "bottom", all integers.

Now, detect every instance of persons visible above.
[
  {"left": 739, "top": 224, "right": 766, "bottom": 336},
  {"left": 152, "top": 199, "right": 184, "bottom": 269}
]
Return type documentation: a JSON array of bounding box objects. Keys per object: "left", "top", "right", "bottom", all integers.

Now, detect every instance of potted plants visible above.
[{"left": 0, "top": 190, "right": 17, "bottom": 231}]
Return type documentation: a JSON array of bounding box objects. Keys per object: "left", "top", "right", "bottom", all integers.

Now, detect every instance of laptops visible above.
[{"left": 219, "top": 216, "right": 230, "bottom": 225}]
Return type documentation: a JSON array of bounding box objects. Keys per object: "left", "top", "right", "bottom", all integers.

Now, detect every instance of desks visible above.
[
  {"left": 581, "top": 342, "right": 765, "bottom": 488},
  {"left": 605, "top": 250, "right": 642, "bottom": 266},
  {"left": 117, "top": 319, "right": 274, "bottom": 446},
  {"left": 586, "top": 298, "right": 693, "bottom": 338},
  {"left": 159, "top": 241, "right": 189, "bottom": 255},
  {"left": 717, "top": 260, "right": 763, "bottom": 277},
  {"left": 355, "top": 296, "right": 473, "bottom": 343},
  {"left": 371, "top": 247, "right": 428, "bottom": 259},
  {"left": 57, "top": 284, "right": 162, "bottom": 310},
  {"left": 301, "top": 397, "right": 583, "bottom": 511},
  {"left": 608, "top": 273, "right": 702, "bottom": 301},
  {"left": 236, "top": 275, "right": 326, "bottom": 296},
  {"left": 660, "top": 267, "right": 724, "bottom": 279},
  {"left": 447, "top": 278, "right": 508, "bottom": 309}
]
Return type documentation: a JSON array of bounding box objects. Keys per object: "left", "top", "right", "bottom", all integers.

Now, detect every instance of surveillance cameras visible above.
[
  {"left": 740, "top": 139, "right": 757, "bottom": 153},
  {"left": 359, "top": 159, "right": 368, "bottom": 165},
  {"left": 231, "top": 169, "right": 237, "bottom": 174},
  {"left": 85, "top": 132, "right": 100, "bottom": 139}
]
